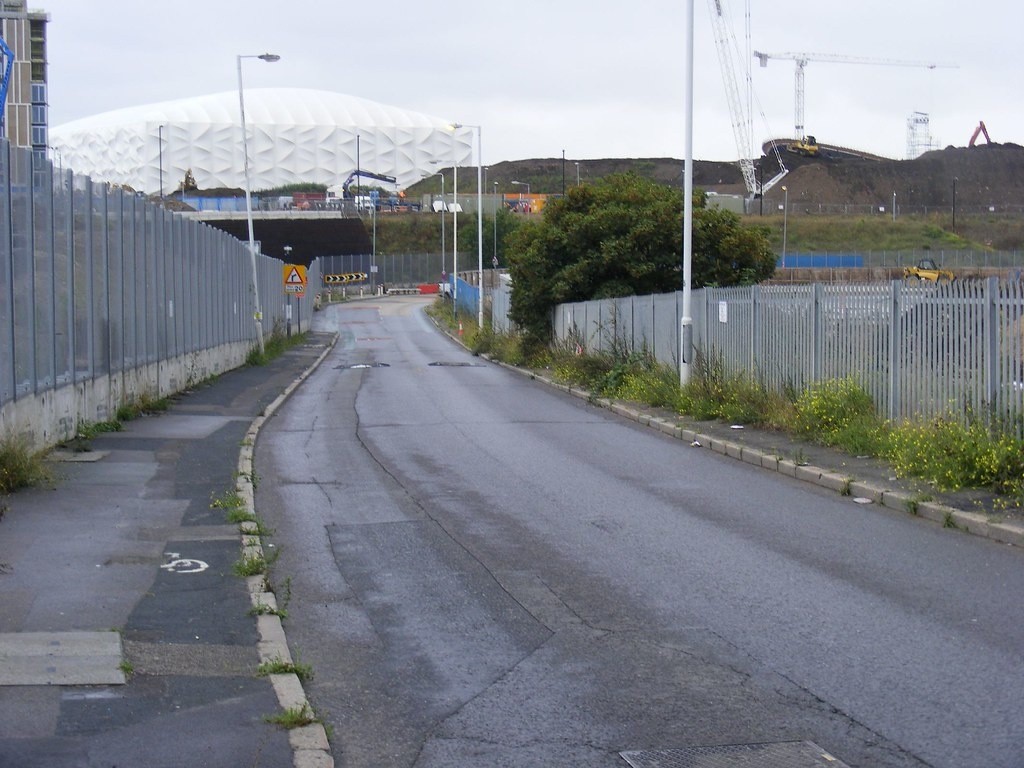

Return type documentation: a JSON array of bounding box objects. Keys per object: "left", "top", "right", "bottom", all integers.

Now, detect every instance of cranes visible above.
[
  {"left": 702, "top": 0, "right": 790, "bottom": 201},
  {"left": 749, "top": 49, "right": 939, "bottom": 143}
]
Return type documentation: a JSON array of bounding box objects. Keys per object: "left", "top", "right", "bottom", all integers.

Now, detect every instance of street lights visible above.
[
  {"left": 429, "top": 159, "right": 457, "bottom": 315},
  {"left": 574, "top": 162, "right": 580, "bottom": 186},
  {"left": 420, "top": 172, "right": 445, "bottom": 301},
  {"left": 510, "top": 179, "right": 530, "bottom": 221},
  {"left": 451, "top": 124, "right": 484, "bottom": 329},
  {"left": 235, "top": 51, "right": 282, "bottom": 356},
  {"left": 492, "top": 181, "right": 499, "bottom": 269},
  {"left": 781, "top": 185, "right": 788, "bottom": 268}
]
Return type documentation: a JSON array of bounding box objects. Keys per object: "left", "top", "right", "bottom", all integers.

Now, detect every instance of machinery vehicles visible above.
[{"left": 342, "top": 170, "right": 422, "bottom": 212}]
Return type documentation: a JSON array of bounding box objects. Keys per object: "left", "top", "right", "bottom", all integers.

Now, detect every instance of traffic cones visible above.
[{"left": 458, "top": 320, "right": 464, "bottom": 335}]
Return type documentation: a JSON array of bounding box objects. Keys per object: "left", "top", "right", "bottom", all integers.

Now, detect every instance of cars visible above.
[{"left": 902, "top": 258, "right": 957, "bottom": 289}]
